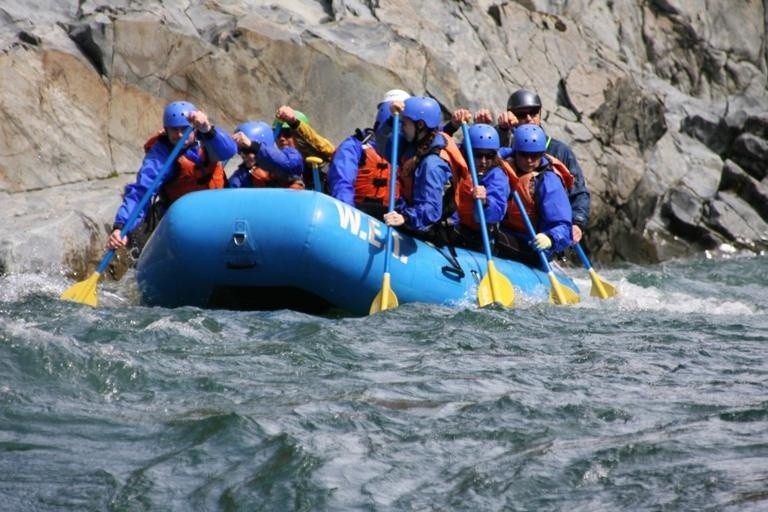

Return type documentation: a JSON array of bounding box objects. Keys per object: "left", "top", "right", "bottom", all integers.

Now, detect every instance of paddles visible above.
[
  {"left": 58, "top": 124, "right": 200, "bottom": 307},
  {"left": 575, "top": 240, "right": 617, "bottom": 300},
  {"left": 461, "top": 118, "right": 515, "bottom": 309},
  {"left": 370, "top": 108, "right": 400, "bottom": 314},
  {"left": 515, "top": 192, "right": 581, "bottom": 309}
]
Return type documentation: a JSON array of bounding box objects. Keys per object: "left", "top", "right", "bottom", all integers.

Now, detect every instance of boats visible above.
[{"left": 129, "top": 183, "right": 587, "bottom": 315}]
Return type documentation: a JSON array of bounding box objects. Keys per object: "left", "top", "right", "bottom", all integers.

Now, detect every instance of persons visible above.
[{"left": 104, "top": 102, "right": 239, "bottom": 251}]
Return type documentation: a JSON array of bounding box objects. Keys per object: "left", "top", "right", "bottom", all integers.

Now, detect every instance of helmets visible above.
[
  {"left": 163, "top": 100, "right": 198, "bottom": 129},
  {"left": 374, "top": 102, "right": 395, "bottom": 133},
  {"left": 402, "top": 96, "right": 442, "bottom": 128},
  {"left": 233, "top": 120, "right": 274, "bottom": 148},
  {"left": 462, "top": 123, "right": 501, "bottom": 151},
  {"left": 507, "top": 89, "right": 542, "bottom": 111},
  {"left": 375, "top": 89, "right": 413, "bottom": 108},
  {"left": 272, "top": 109, "right": 310, "bottom": 129},
  {"left": 511, "top": 124, "right": 547, "bottom": 154}
]
